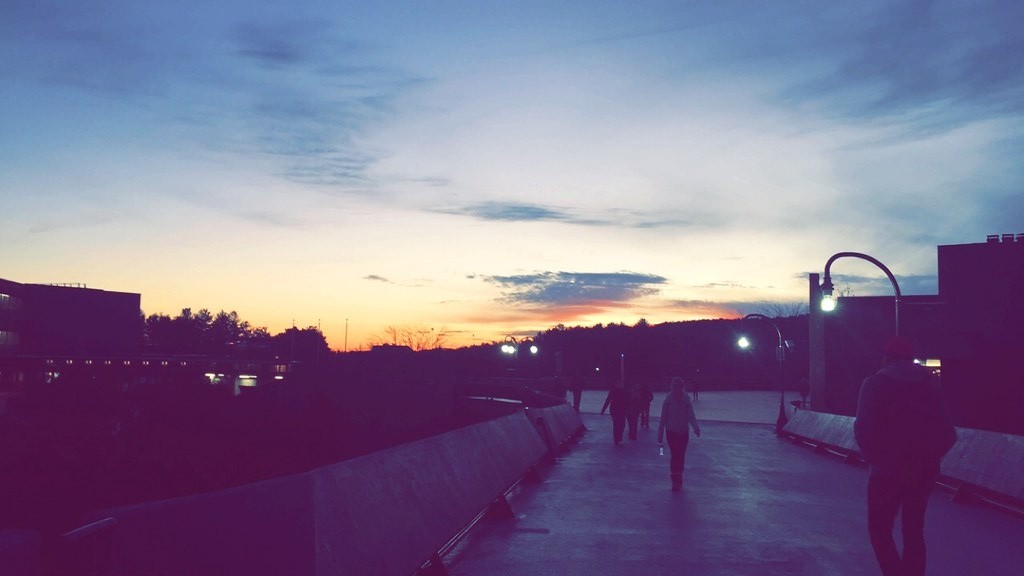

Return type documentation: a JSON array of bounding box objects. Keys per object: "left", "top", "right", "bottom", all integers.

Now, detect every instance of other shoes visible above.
[{"left": 672, "top": 481, "right": 680, "bottom": 491}]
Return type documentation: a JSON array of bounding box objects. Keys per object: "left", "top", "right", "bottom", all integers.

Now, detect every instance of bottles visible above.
[{"left": 659, "top": 446, "right": 664, "bottom": 456}]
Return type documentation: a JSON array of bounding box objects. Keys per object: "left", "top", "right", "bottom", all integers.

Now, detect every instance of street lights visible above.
[
  {"left": 502, "top": 334, "right": 539, "bottom": 381},
  {"left": 737, "top": 313, "right": 788, "bottom": 433},
  {"left": 818, "top": 251, "right": 901, "bottom": 352}
]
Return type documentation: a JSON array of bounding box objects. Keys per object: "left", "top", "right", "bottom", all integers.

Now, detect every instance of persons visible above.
[
  {"left": 854, "top": 336, "right": 958, "bottom": 576},
  {"left": 569, "top": 370, "right": 584, "bottom": 411},
  {"left": 799, "top": 377, "right": 809, "bottom": 408},
  {"left": 691, "top": 380, "right": 700, "bottom": 400},
  {"left": 657, "top": 376, "right": 701, "bottom": 492},
  {"left": 598, "top": 378, "right": 654, "bottom": 445}
]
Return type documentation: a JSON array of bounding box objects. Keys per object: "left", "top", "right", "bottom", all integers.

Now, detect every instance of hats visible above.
[{"left": 879, "top": 336, "right": 919, "bottom": 357}]
